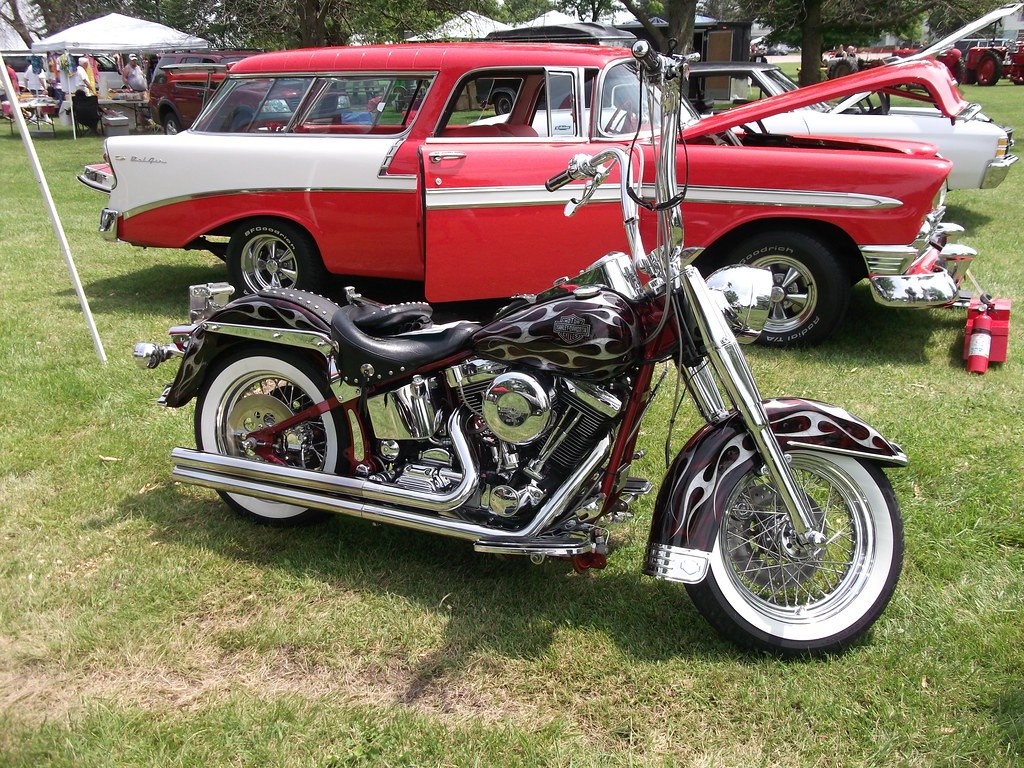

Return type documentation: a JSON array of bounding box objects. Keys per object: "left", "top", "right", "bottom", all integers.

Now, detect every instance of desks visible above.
[
  {"left": 2, "top": 101, "right": 56, "bottom": 137},
  {"left": 58, "top": 99, "right": 149, "bottom": 138}
]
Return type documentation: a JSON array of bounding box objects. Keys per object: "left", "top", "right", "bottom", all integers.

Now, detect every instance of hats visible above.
[{"left": 129, "top": 54, "right": 137, "bottom": 60}]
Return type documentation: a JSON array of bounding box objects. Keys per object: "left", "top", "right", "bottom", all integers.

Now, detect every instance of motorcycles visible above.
[{"left": 133, "top": 1, "right": 910, "bottom": 661}]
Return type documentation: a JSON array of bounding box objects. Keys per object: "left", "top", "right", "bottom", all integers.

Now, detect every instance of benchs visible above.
[{"left": 290, "top": 124, "right": 408, "bottom": 135}]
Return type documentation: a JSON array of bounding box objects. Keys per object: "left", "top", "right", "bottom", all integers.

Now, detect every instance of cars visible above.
[
  {"left": 463, "top": 22, "right": 1019, "bottom": 192},
  {"left": 77, "top": 39, "right": 993, "bottom": 351},
  {"left": 664, "top": 58, "right": 832, "bottom": 125}
]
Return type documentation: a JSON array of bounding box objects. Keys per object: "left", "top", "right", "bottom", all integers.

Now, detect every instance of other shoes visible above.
[
  {"left": 29, "top": 118, "right": 38, "bottom": 125},
  {"left": 43, "top": 121, "right": 54, "bottom": 125}
]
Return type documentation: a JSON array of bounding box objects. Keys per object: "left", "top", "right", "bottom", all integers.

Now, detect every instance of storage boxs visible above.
[{"left": 102, "top": 115, "right": 130, "bottom": 137}]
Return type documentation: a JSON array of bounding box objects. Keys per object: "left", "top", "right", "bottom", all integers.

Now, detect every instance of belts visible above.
[{"left": 133, "top": 90, "right": 146, "bottom": 92}]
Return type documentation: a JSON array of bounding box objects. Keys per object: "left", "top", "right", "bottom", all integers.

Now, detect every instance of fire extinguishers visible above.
[{"left": 966, "top": 292, "right": 998, "bottom": 376}]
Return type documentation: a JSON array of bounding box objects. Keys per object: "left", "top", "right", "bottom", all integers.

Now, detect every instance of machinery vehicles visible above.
[{"left": 826, "top": 36, "right": 1024, "bottom": 91}]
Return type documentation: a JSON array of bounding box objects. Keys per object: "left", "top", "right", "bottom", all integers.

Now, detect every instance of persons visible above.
[{"left": 3, "top": 53, "right": 147, "bottom": 133}]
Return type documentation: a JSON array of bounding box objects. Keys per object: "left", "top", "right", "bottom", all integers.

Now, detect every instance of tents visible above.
[
  {"left": 32, "top": 13, "right": 208, "bottom": 98},
  {"left": 403, "top": 0, "right": 774, "bottom": 47}
]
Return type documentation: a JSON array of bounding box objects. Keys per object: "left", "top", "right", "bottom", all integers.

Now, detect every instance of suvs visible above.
[{"left": 150, "top": 48, "right": 352, "bottom": 135}]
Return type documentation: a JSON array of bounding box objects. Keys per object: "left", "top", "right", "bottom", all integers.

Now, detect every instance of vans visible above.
[{"left": 0, "top": 48, "right": 130, "bottom": 120}]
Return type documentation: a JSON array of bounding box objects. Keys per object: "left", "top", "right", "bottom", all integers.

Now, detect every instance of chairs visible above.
[
  {"left": 393, "top": 85, "right": 413, "bottom": 111},
  {"left": 439, "top": 124, "right": 537, "bottom": 138}
]
